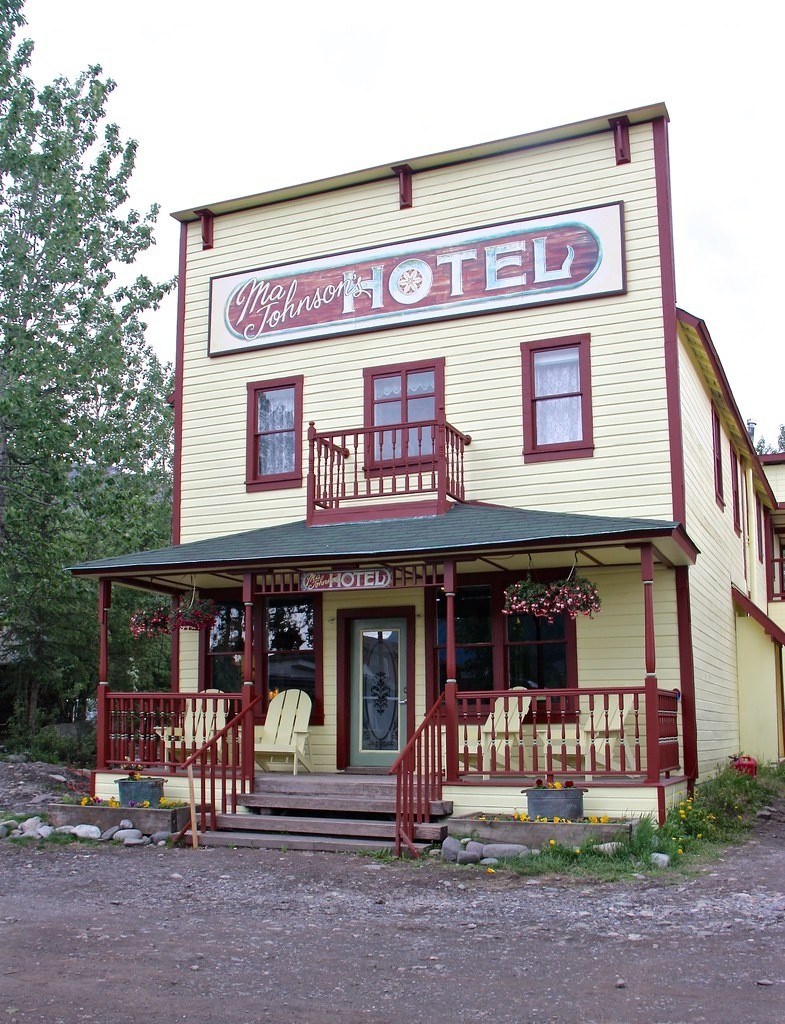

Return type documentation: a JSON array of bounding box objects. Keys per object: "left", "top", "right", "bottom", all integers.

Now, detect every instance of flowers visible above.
[
  {"left": 129, "top": 606, "right": 169, "bottom": 640},
  {"left": 535, "top": 779, "right": 574, "bottom": 789},
  {"left": 479, "top": 812, "right": 609, "bottom": 824},
  {"left": 62, "top": 791, "right": 186, "bottom": 810},
  {"left": 546, "top": 576, "right": 602, "bottom": 625},
  {"left": 169, "top": 595, "right": 219, "bottom": 632},
  {"left": 501, "top": 571, "right": 549, "bottom": 617},
  {"left": 125, "top": 764, "right": 145, "bottom": 781}
]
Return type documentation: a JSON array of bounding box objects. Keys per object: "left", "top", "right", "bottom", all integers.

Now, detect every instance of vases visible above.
[
  {"left": 447, "top": 810, "right": 643, "bottom": 848},
  {"left": 49, "top": 801, "right": 191, "bottom": 835},
  {"left": 521, "top": 787, "right": 588, "bottom": 821},
  {"left": 113, "top": 777, "right": 169, "bottom": 806}
]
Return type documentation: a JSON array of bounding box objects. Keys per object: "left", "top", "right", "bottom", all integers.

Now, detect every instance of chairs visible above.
[
  {"left": 153, "top": 687, "right": 232, "bottom": 772},
  {"left": 537, "top": 693, "right": 640, "bottom": 782},
  {"left": 237, "top": 689, "right": 312, "bottom": 776},
  {"left": 440, "top": 686, "right": 531, "bottom": 781}
]
